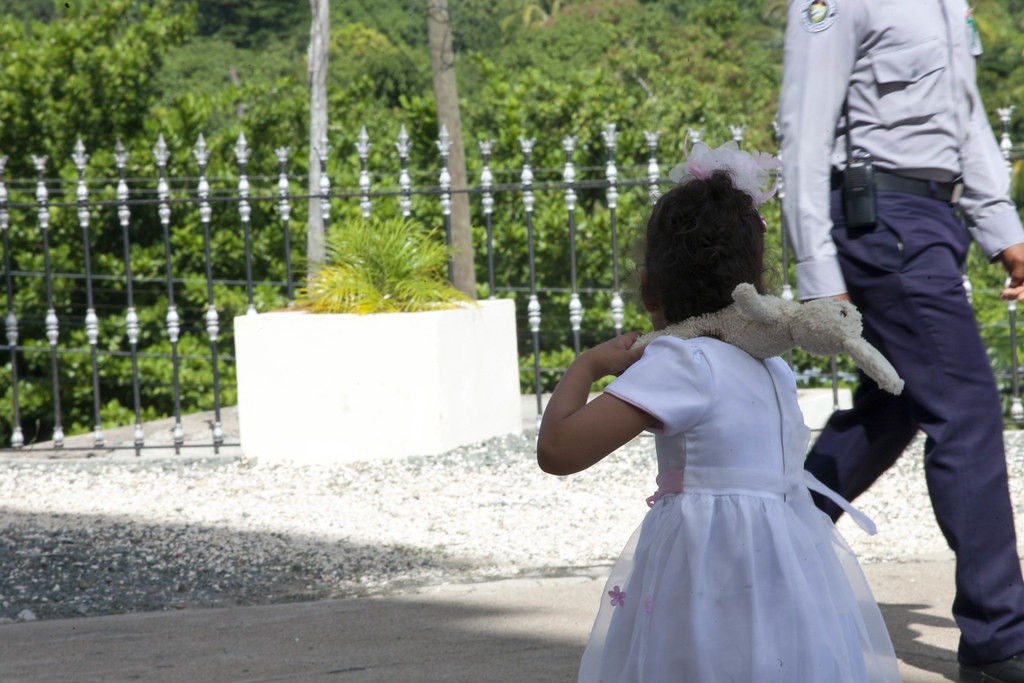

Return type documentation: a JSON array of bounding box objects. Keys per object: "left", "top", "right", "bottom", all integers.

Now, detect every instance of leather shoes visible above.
[{"left": 960, "top": 654, "right": 1024, "bottom": 683}]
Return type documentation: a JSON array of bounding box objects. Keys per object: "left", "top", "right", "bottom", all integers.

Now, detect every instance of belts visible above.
[{"left": 874, "top": 173, "right": 964, "bottom": 203}]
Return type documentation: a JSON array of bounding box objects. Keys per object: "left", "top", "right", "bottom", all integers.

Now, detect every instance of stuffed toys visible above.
[{"left": 625, "top": 280, "right": 905, "bottom": 397}]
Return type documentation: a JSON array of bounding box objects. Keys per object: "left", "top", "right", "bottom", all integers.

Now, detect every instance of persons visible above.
[
  {"left": 536, "top": 172, "right": 873, "bottom": 683},
  {"left": 782, "top": 0, "right": 1024, "bottom": 683}
]
